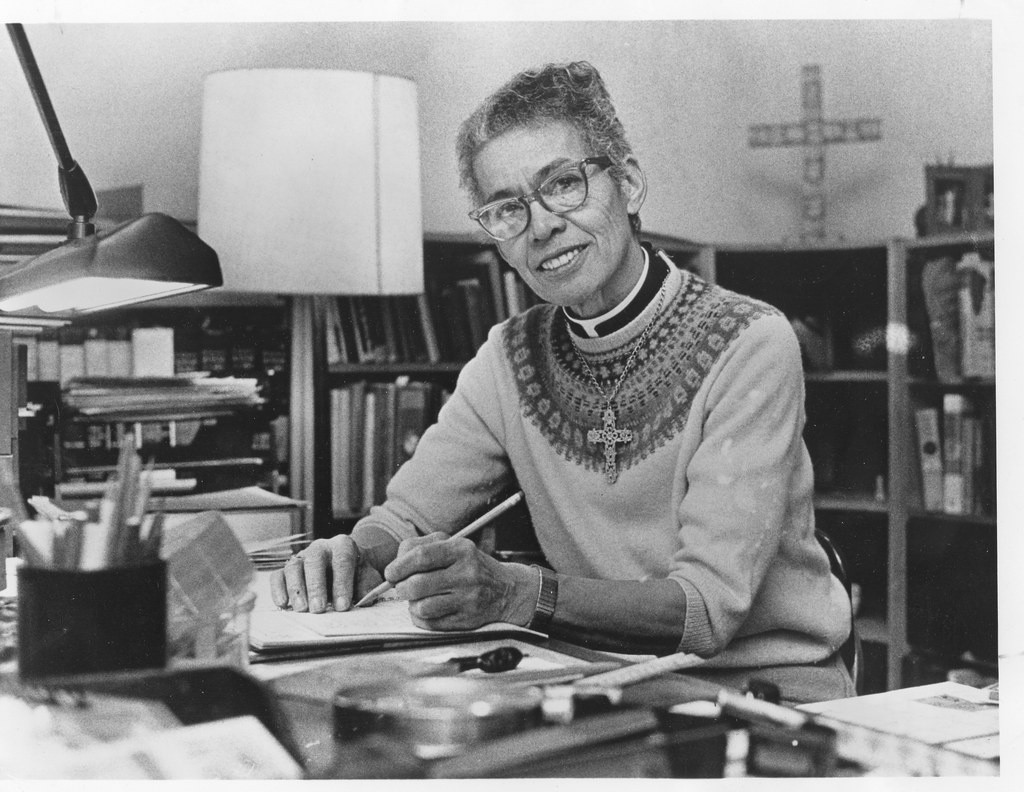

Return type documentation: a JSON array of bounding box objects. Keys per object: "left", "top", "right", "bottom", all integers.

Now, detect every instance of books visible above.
[
  {"left": 0, "top": 247, "right": 518, "bottom": 666},
  {"left": 916, "top": 254, "right": 997, "bottom": 524}
]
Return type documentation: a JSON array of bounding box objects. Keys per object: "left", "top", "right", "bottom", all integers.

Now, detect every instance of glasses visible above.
[{"left": 468, "top": 157, "right": 612, "bottom": 241}]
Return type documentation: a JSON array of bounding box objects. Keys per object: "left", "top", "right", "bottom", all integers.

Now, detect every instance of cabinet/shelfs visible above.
[{"left": 0, "top": 214, "right": 997, "bottom": 698}]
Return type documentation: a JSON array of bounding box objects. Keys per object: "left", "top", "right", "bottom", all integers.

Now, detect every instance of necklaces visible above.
[{"left": 564, "top": 278, "right": 667, "bottom": 483}]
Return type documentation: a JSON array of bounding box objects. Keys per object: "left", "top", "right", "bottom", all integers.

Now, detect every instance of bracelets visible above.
[{"left": 526, "top": 564, "right": 559, "bottom": 634}]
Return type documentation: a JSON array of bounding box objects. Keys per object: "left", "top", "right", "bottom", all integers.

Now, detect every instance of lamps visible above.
[
  {"left": 0, "top": 23, "right": 222, "bottom": 315},
  {"left": 197, "top": 69, "right": 423, "bottom": 540}
]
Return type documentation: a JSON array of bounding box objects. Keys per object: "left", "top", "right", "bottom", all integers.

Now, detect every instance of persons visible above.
[{"left": 270, "top": 61, "right": 858, "bottom": 707}]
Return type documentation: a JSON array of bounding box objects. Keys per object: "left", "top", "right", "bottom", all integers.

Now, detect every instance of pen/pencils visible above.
[
  {"left": 23, "top": 430, "right": 167, "bottom": 573},
  {"left": 354, "top": 491, "right": 525, "bottom": 607}
]
